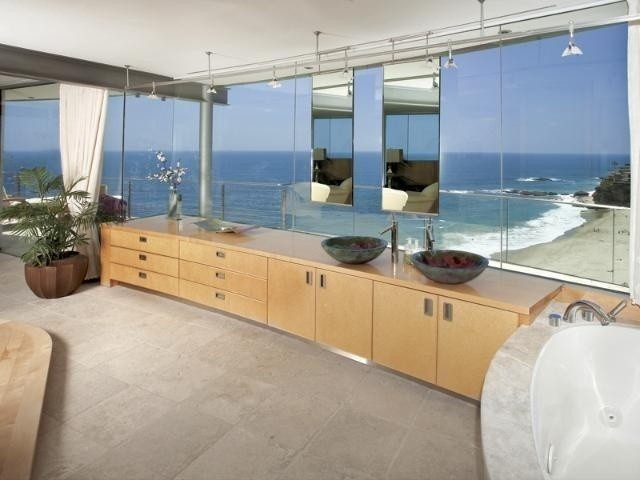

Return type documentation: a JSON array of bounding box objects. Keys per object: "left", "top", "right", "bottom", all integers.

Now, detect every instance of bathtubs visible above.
[{"left": 477, "top": 312, "right": 640, "bottom": 480}]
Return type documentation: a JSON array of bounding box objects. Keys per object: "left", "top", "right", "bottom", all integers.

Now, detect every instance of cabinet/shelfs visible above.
[{"left": 97, "top": 224, "right": 565, "bottom": 406}]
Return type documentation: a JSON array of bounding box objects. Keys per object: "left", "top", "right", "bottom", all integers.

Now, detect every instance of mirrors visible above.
[
  {"left": 381, "top": 55, "right": 442, "bottom": 216},
  {"left": 311, "top": 68, "right": 355, "bottom": 211}
]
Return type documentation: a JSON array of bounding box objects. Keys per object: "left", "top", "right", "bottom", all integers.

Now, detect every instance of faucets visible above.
[
  {"left": 423, "top": 223, "right": 436, "bottom": 250},
  {"left": 390, "top": 244, "right": 399, "bottom": 264},
  {"left": 562, "top": 298, "right": 611, "bottom": 327},
  {"left": 379, "top": 219, "right": 399, "bottom": 244}
]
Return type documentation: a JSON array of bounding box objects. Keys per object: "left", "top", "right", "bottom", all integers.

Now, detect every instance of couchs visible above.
[
  {"left": 312, "top": 175, "right": 352, "bottom": 204},
  {"left": 382, "top": 180, "right": 439, "bottom": 214}
]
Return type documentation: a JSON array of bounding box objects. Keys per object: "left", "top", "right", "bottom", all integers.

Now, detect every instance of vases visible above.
[{"left": 165, "top": 188, "right": 184, "bottom": 222}]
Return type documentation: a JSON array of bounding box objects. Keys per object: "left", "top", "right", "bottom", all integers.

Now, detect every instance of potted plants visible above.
[{"left": 1, "top": 167, "right": 125, "bottom": 299}]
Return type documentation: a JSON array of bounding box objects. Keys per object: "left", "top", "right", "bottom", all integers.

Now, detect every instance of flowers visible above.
[{"left": 143, "top": 148, "right": 191, "bottom": 216}]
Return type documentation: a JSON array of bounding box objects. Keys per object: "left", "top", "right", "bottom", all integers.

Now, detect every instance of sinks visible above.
[
  {"left": 320, "top": 234, "right": 388, "bottom": 265},
  {"left": 410, "top": 249, "right": 489, "bottom": 286}
]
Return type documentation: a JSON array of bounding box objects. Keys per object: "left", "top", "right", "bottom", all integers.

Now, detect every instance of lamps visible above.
[
  {"left": 443, "top": 40, "right": 460, "bottom": 73},
  {"left": 267, "top": 64, "right": 282, "bottom": 89},
  {"left": 314, "top": 148, "right": 328, "bottom": 172},
  {"left": 385, "top": 148, "right": 403, "bottom": 173},
  {"left": 147, "top": 78, "right": 161, "bottom": 100},
  {"left": 561, "top": 19, "right": 583, "bottom": 59},
  {"left": 205, "top": 73, "right": 217, "bottom": 95}
]
